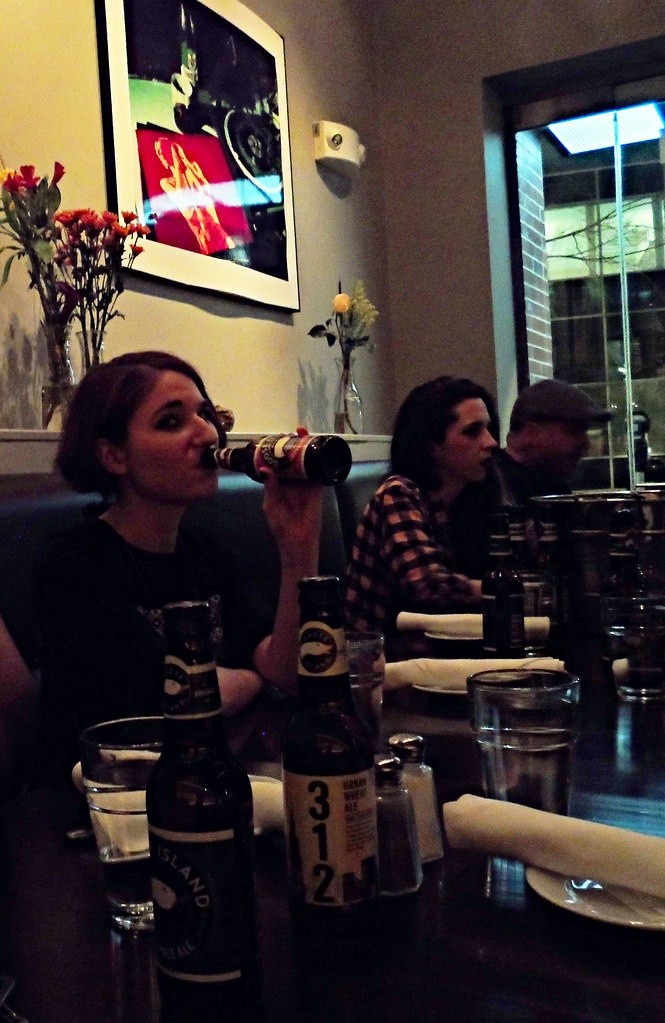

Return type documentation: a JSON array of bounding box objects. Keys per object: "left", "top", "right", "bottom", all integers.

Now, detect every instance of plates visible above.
[
  {"left": 424, "top": 631, "right": 483, "bottom": 640},
  {"left": 526, "top": 863, "right": 665, "bottom": 927},
  {"left": 411, "top": 683, "right": 468, "bottom": 695}
]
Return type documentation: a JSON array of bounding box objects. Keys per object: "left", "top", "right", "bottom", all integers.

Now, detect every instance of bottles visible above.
[
  {"left": 389, "top": 731, "right": 444, "bottom": 863},
  {"left": 601, "top": 510, "right": 647, "bottom": 658},
  {"left": 375, "top": 754, "right": 424, "bottom": 896},
  {"left": 155, "top": 602, "right": 256, "bottom": 983},
  {"left": 483, "top": 514, "right": 526, "bottom": 659},
  {"left": 509, "top": 517, "right": 563, "bottom": 625},
  {"left": 282, "top": 576, "right": 388, "bottom": 928},
  {"left": 204, "top": 434, "right": 353, "bottom": 485}
]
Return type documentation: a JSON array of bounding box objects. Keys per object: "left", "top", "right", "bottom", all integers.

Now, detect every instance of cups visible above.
[
  {"left": 516, "top": 570, "right": 556, "bottom": 653},
  {"left": 79, "top": 716, "right": 157, "bottom": 934},
  {"left": 603, "top": 591, "right": 647, "bottom": 665},
  {"left": 612, "top": 608, "right": 665, "bottom": 703},
  {"left": 345, "top": 630, "right": 387, "bottom": 746},
  {"left": 468, "top": 669, "right": 580, "bottom": 816}
]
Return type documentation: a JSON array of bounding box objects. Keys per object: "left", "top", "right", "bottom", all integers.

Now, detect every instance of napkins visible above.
[
  {"left": 442, "top": 793, "right": 665, "bottom": 899},
  {"left": 394, "top": 610, "right": 550, "bottom": 640},
  {"left": 71, "top": 749, "right": 282, "bottom": 831},
  {"left": 381, "top": 657, "right": 567, "bottom": 690}
]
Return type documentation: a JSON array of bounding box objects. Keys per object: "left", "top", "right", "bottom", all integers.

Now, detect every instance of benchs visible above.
[{"left": 0, "top": 461, "right": 390, "bottom": 668}]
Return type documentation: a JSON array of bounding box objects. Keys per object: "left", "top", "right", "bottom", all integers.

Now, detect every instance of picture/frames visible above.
[{"left": 94, "top": 0, "right": 300, "bottom": 312}]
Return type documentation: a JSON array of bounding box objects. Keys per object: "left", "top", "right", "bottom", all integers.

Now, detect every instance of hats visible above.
[{"left": 511, "top": 380, "right": 614, "bottom": 422}]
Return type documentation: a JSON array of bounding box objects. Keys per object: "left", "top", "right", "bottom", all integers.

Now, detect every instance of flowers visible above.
[
  {"left": 304, "top": 277, "right": 381, "bottom": 433},
  {"left": 0, "top": 160, "right": 152, "bottom": 405}
]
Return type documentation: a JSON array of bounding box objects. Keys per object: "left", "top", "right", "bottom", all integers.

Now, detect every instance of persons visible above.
[
  {"left": 343, "top": 378, "right": 550, "bottom": 646},
  {"left": 32, "top": 352, "right": 327, "bottom": 726},
  {"left": 449, "top": 380, "right": 614, "bottom": 579}
]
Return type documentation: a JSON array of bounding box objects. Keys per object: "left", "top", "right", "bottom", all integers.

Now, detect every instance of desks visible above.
[{"left": 0, "top": 618, "right": 665, "bottom": 1023}]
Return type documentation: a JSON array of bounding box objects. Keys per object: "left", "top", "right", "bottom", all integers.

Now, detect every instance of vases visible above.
[
  {"left": 74, "top": 330, "right": 107, "bottom": 376},
  {"left": 36, "top": 323, "right": 80, "bottom": 430},
  {"left": 333, "top": 356, "right": 364, "bottom": 434}
]
